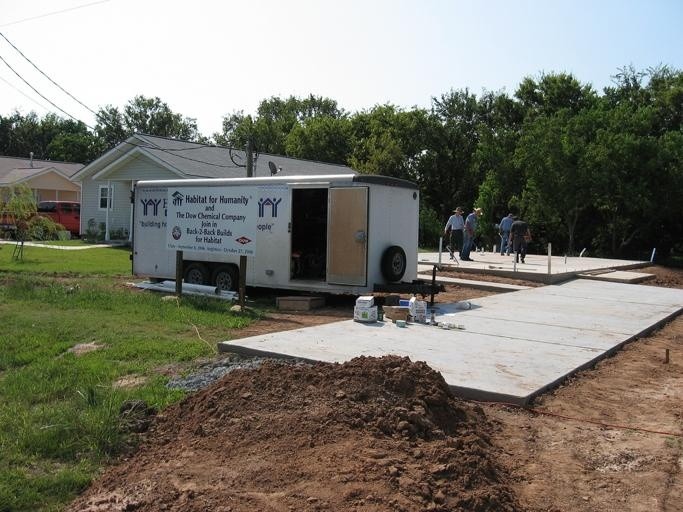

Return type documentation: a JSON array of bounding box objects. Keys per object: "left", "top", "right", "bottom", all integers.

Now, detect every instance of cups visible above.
[{"left": 439, "top": 321, "right": 464, "bottom": 329}]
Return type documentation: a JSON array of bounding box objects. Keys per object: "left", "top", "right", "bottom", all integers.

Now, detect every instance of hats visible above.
[
  {"left": 473, "top": 208, "right": 484, "bottom": 216},
  {"left": 453, "top": 207, "right": 464, "bottom": 214}
]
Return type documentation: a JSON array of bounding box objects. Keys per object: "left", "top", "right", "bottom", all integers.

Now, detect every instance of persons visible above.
[
  {"left": 444, "top": 206, "right": 466, "bottom": 260},
  {"left": 497, "top": 213, "right": 514, "bottom": 256},
  {"left": 460, "top": 207, "right": 485, "bottom": 261},
  {"left": 507, "top": 215, "right": 532, "bottom": 264}
]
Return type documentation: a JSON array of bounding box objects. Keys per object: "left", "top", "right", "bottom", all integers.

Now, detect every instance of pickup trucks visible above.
[{"left": 0, "top": 198, "right": 80, "bottom": 241}]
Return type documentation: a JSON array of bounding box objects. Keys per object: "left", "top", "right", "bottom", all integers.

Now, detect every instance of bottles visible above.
[
  {"left": 430, "top": 309, "right": 436, "bottom": 325},
  {"left": 409, "top": 315, "right": 414, "bottom": 321},
  {"left": 378, "top": 311, "right": 383, "bottom": 321}
]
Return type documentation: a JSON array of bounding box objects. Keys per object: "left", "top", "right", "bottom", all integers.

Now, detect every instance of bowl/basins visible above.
[{"left": 395, "top": 320, "right": 406, "bottom": 328}]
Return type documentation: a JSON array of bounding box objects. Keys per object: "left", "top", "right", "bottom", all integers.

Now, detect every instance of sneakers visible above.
[
  {"left": 512, "top": 258, "right": 524, "bottom": 263},
  {"left": 450, "top": 255, "right": 474, "bottom": 261}
]
